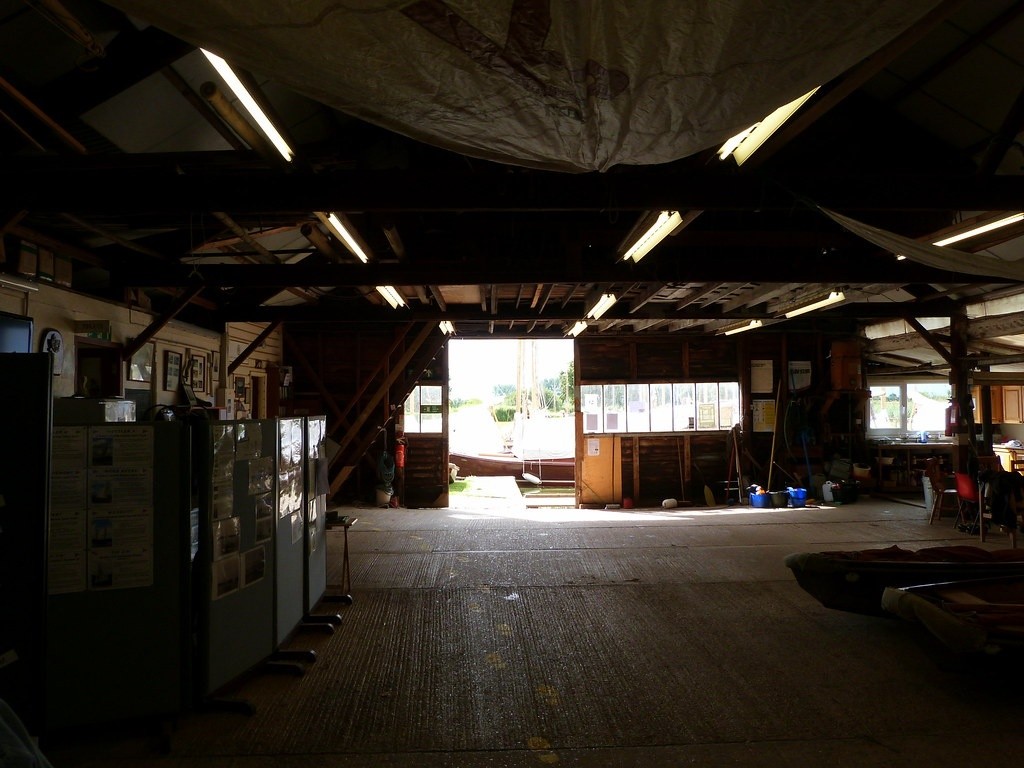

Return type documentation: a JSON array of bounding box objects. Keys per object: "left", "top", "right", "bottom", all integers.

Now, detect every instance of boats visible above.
[
  {"left": 449, "top": 453, "right": 575, "bottom": 484},
  {"left": 783, "top": 545, "right": 1024, "bottom": 671}
]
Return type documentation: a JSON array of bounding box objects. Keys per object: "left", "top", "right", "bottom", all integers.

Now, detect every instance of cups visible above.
[{"left": 662, "top": 498, "right": 678, "bottom": 508}]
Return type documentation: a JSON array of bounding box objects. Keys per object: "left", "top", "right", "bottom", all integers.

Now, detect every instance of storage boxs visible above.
[
  {"left": 890, "top": 473, "right": 897, "bottom": 480},
  {"left": 875, "top": 457, "right": 895, "bottom": 464},
  {"left": 716, "top": 481, "right": 739, "bottom": 504},
  {"left": 897, "top": 472, "right": 903, "bottom": 486},
  {"left": 883, "top": 481, "right": 896, "bottom": 487}
]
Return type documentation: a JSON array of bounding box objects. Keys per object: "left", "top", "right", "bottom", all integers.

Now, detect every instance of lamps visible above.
[
  {"left": 716, "top": 86, "right": 822, "bottom": 168},
  {"left": 584, "top": 290, "right": 618, "bottom": 321},
  {"left": 325, "top": 212, "right": 370, "bottom": 265},
  {"left": 617, "top": 210, "right": 684, "bottom": 264},
  {"left": 715, "top": 319, "right": 763, "bottom": 336},
  {"left": 897, "top": 211, "right": 1024, "bottom": 261},
  {"left": 779, "top": 290, "right": 846, "bottom": 319},
  {"left": 563, "top": 320, "right": 588, "bottom": 338},
  {"left": 201, "top": 48, "right": 296, "bottom": 164},
  {"left": 376, "top": 285, "right": 410, "bottom": 310},
  {"left": 439, "top": 321, "right": 457, "bottom": 336}
]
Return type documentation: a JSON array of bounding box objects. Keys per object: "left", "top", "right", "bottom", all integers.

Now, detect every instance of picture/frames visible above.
[
  {"left": 191, "top": 355, "right": 205, "bottom": 392},
  {"left": 163, "top": 349, "right": 183, "bottom": 393}
]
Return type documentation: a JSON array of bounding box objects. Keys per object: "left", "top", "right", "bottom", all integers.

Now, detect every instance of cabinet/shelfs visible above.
[
  {"left": 878, "top": 445, "right": 954, "bottom": 493},
  {"left": 970, "top": 385, "right": 1003, "bottom": 424},
  {"left": 1001, "top": 385, "right": 1024, "bottom": 424}
]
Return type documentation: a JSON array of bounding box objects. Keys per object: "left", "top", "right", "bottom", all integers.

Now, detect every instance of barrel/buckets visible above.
[
  {"left": 751, "top": 491, "right": 770, "bottom": 508},
  {"left": 787, "top": 488, "right": 807, "bottom": 507},
  {"left": 823, "top": 481, "right": 838, "bottom": 502},
  {"left": 853, "top": 463, "right": 871, "bottom": 484},
  {"left": 769, "top": 490, "right": 789, "bottom": 508},
  {"left": 376, "top": 486, "right": 393, "bottom": 506},
  {"left": 623, "top": 497, "right": 633, "bottom": 508}
]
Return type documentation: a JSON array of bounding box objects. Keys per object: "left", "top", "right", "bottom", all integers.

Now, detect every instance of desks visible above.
[
  {"left": 993, "top": 448, "right": 1024, "bottom": 476},
  {"left": 326, "top": 518, "right": 359, "bottom": 595}
]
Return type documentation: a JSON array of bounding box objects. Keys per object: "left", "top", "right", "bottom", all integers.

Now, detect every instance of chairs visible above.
[
  {"left": 953, "top": 471, "right": 987, "bottom": 535},
  {"left": 924, "top": 456, "right": 975, "bottom": 525},
  {"left": 977, "top": 473, "right": 1024, "bottom": 549},
  {"left": 976, "top": 456, "right": 999, "bottom": 472},
  {"left": 1011, "top": 460, "right": 1024, "bottom": 472}
]
